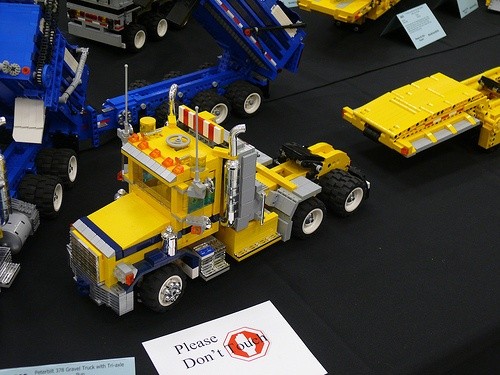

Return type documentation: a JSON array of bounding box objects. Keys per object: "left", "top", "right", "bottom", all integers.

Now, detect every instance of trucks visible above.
[
  {"left": 0, "top": 0, "right": 309, "bottom": 289},
  {"left": 66, "top": 0, "right": 198, "bottom": 52},
  {"left": 66, "top": 65, "right": 371, "bottom": 318},
  {"left": 342, "top": 64, "right": 500, "bottom": 158},
  {"left": 297, "top": 0, "right": 402, "bottom": 31}
]
